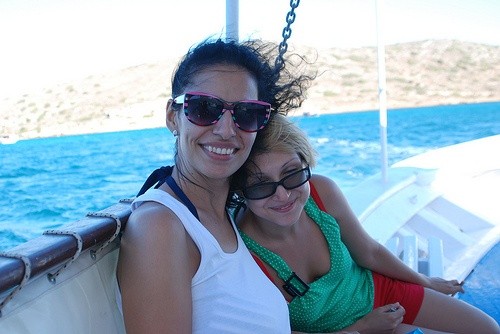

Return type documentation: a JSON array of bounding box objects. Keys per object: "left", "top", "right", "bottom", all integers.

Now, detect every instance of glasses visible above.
[
  {"left": 240, "top": 164, "right": 311, "bottom": 199},
  {"left": 173, "top": 91, "right": 276, "bottom": 132}
]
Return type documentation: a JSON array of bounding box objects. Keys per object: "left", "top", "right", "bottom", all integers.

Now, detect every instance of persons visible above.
[
  {"left": 114, "top": 37, "right": 320, "bottom": 334},
  {"left": 227, "top": 113, "right": 500, "bottom": 334}
]
